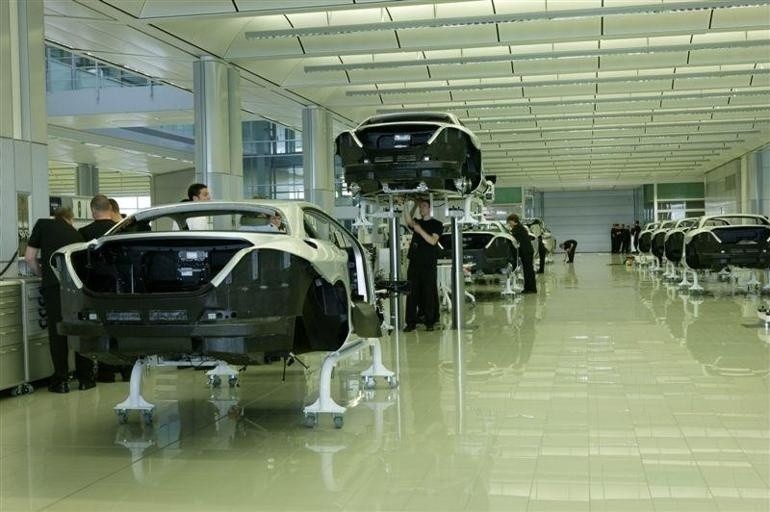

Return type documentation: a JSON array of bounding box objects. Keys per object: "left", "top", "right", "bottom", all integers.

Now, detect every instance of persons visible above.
[
  {"left": 397, "top": 196, "right": 444, "bottom": 332},
  {"left": 538, "top": 235, "right": 547, "bottom": 274},
  {"left": 78, "top": 194, "right": 133, "bottom": 383},
  {"left": 109, "top": 198, "right": 153, "bottom": 232},
  {"left": 24, "top": 205, "right": 97, "bottom": 392},
  {"left": 267, "top": 212, "right": 286, "bottom": 230},
  {"left": 559, "top": 240, "right": 577, "bottom": 263},
  {"left": 171, "top": 183, "right": 212, "bottom": 231},
  {"left": 506, "top": 214, "right": 537, "bottom": 294},
  {"left": 611, "top": 220, "right": 641, "bottom": 254}
]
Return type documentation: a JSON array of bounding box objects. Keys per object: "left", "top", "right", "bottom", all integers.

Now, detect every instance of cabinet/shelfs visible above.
[{"left": 0, "top": 275, "right": 76, "bottom": 390}]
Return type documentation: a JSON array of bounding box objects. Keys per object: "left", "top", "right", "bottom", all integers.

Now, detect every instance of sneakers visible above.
[
  {"left": 47, "top": 382, "right": 70, "bottom": 393},
  {"left": 79, "top": 380, "right": 96, "bottom": 390},
  {"left": 402, "top": 324, "right": 416, "bottom": 331},
  {"left": 426, "top": 327, "right": 433, "bottom": 331}
]
